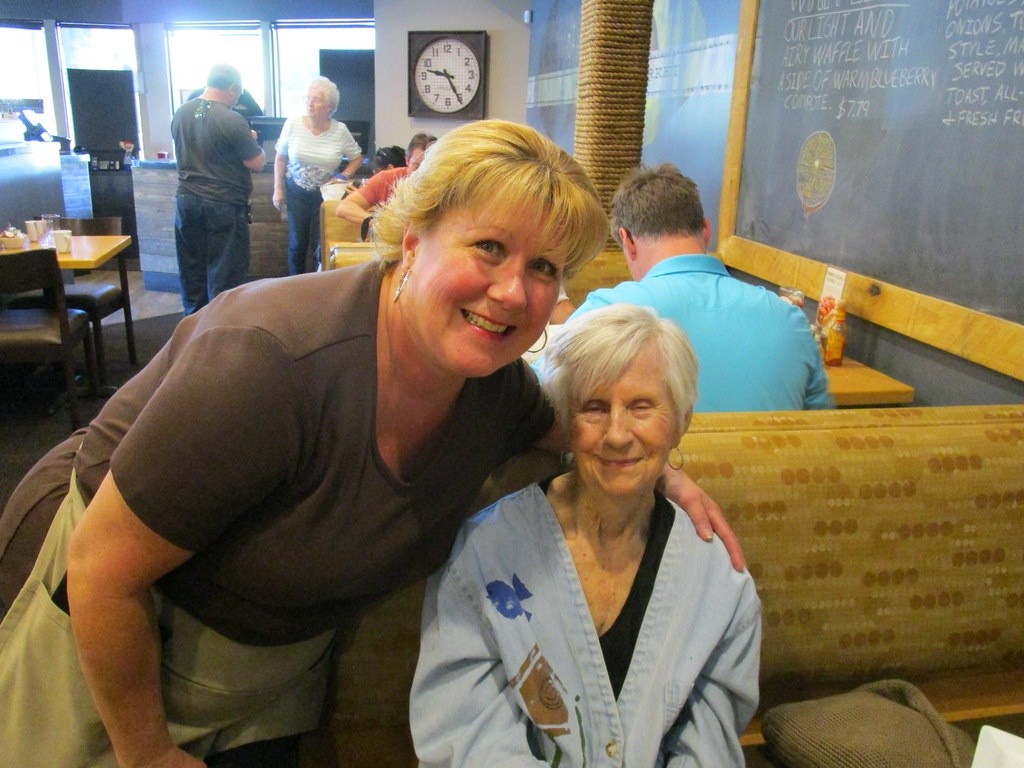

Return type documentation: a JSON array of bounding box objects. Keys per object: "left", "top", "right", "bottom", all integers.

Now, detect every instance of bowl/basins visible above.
[{"left": 0, "top": 234, "right": 27, "bottom": 248}]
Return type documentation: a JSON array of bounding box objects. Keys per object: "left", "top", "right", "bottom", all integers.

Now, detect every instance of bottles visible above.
[{"left": 825, "top": 300, "right": 847, "bottom": 366}]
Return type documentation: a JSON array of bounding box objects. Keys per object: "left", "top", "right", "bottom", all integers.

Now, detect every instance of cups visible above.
[
  {"left": 25, "top": 221, "right": 44, "bottom": 241},
  {"left": 54, "top": 229, "right": 73, "bottom": 254},
  {"left": 34, "top": 220, "right": 54, "bottom": 247},
  {"left": 42, "top": 214, "right": 60, "bottom": 230}
]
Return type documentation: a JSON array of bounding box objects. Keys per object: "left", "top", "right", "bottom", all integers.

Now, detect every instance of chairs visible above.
[
  {"left": 5, "top": 216, "right": 138, "bottom": 382},
  {"left": 0, "top": 247, "right": 99, "bottom": 428}
]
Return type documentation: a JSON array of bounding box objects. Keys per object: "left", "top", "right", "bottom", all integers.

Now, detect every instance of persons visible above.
[
  {"left": 535, "top": 160, "right": 835, "bottom": 414},
  {"left": 168, "top": 67, "right": 268, "bottom": 318},
  {"left": 345, "top": 144, "right": 405, "bottom": 199},
  {"left": 189, "top": 86, "right": 262, "bottom": 117},
  {"left": 271, "top": 73, "right": 366, "bottom": 274},
  {"left": 407, "top": 304, "right": 762, "bottom": 766},
  {"left": 0, "top": 116, "right": 748, "bottom": 768},
  {"left": 337, "top": 132, "right": 440, "bottom": 243}
]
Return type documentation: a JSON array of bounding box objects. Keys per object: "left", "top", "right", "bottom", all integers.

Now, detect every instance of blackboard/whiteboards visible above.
[{"left": 718, "top": 1, "right": 1023, "bottom": 386}]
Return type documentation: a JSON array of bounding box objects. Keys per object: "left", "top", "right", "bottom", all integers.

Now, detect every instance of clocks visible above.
[{"left": 407, "top": 30, "right": 489, "bottom": 120}]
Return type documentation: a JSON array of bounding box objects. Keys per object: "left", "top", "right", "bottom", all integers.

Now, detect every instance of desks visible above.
[
  {"left": 0, "top": 235, "right": 139, "bottom": 396},
  {"left": 823, "top": 354, "right": 914, "bottom": 406},
  {"left": 328, "top": 243, "right": 390, "bottom": 269}
]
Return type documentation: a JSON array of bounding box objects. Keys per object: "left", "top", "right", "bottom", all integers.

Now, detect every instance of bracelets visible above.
[
  {"left": 361, "top": 216, "right": 373, "bottom": 240},
  {"left": 335, "top": 172, "right": 347, "bottom": 180}
]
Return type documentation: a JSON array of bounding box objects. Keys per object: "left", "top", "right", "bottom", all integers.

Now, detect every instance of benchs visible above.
[{"left": 326, "top": 399, "right": 1024, "bottom": 768}]
